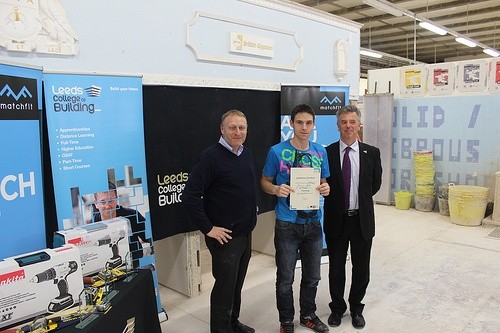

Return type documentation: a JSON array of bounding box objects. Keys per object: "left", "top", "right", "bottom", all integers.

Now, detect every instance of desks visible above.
[{"left": 0, "top": 269, "right": 164, "bottom": 333}]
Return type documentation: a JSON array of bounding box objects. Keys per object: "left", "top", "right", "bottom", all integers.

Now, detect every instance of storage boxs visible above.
[
  {"left": 53, "top": 217, "right": 132, "bottom": 275},
  {"left": 0, "top": 245, "right": 88, "bottom": 327}
]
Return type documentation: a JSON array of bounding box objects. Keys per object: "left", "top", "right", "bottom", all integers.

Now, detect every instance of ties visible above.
[{"left": 341, "top": 147, "right": 352, "bottom": 211}]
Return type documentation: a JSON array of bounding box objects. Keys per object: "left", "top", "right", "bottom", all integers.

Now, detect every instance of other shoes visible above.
[{"left": 232, "top": 321, "right": 255, "bottom": 333}]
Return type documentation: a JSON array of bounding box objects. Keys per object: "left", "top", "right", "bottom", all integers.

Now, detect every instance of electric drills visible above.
[
  {"left": 94, "top": 230, "right": 126, "bottom": 270},
  {"left": 29, "top": 261, "right": 78, "bottom": 311}
]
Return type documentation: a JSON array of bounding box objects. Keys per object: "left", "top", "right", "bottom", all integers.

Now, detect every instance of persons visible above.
[
  {"left": 324, "top": 105, "right": 382, "bottom": 329},
  {"left": 90, "top": 181, "right": 154, "bottom": 270},
  {"left": 182, "top": 110, "right": 257, "bottom": 333},
  {"left": 261, "top": 105, "right": 330, "bottom": 333}
]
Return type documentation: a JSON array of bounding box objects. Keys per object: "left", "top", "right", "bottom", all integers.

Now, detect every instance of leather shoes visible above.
[
  {"left": 328, "top": 312, "right": 343, "bottom": 327},
  {"left": 351, "top": 312, "right": 365, "bottom": 329}
]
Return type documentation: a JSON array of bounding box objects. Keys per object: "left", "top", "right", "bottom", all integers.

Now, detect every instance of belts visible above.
[{"left": 347, "top": 210, "right": 359, "bottom": 216}]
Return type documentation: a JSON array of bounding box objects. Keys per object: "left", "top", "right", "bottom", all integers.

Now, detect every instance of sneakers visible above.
[
  {"left": 280, "top": 322, "right": 294, "bottom": 333},
  {"left": 300, "top": 314, "right": 329, "bottom": 333}
]
